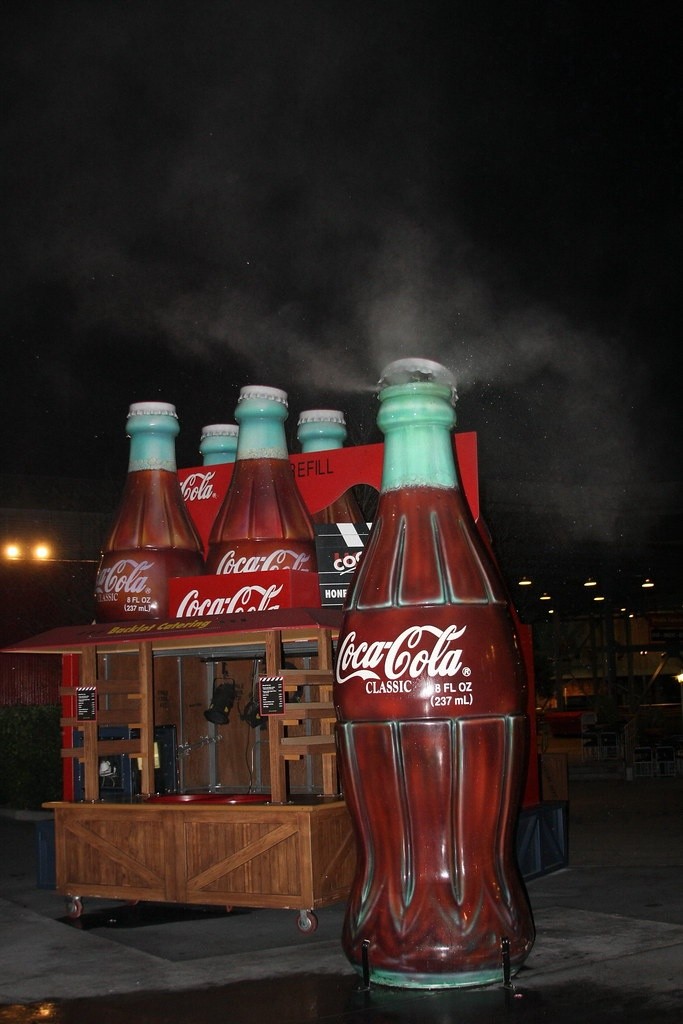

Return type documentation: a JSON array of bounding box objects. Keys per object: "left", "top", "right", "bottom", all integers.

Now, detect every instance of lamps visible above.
[{"left": 202, "top": 675, "right": 269, "bottom": 728}]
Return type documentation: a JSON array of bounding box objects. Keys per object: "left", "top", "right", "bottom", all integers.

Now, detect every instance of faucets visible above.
[{"left": 176, "top": 734, "right": 222, "bottom": 759}]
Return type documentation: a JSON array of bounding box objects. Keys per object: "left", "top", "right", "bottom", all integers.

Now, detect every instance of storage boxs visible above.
[{"left": 541, "top": 715, "right": 683, "bottom": 803}]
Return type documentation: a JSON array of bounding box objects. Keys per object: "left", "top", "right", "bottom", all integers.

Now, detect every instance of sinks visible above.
[
  {"left": 226, "top": 794, "right": 270, "bottom": 801},
  {"left": 148, "top": 792, "right": 206, "bottom": 801}
]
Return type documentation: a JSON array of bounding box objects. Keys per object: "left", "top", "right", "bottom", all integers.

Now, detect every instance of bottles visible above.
[
  {"left": 297, "top": 409, "right": 367, "bottom": 533},
  {"left": 93, "top": 402, "right": 204, "bottom": 622},
  {"left": 334, "top": 357, "right": 531, "bottom": 989},
  {"left": 207, "top": 384, "right": 319, "bottom": 574},
  {"left": 198, "top": 423, "right": 239, "bottom": 466}
]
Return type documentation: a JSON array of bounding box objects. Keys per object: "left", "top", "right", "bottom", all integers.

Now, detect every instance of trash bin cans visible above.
[{"left": 35, "top": 818, "right": 57, "bottom": 889}]
